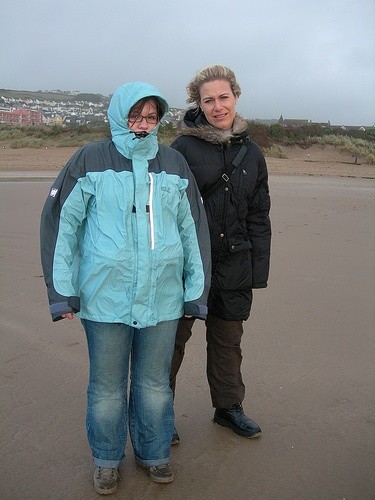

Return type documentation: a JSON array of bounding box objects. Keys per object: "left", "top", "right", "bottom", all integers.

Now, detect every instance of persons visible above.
[
  {"left": 167, "top": 64, "right": 273, "bottom": 445},
  {"left": 38, "top": 80, "right": 212, "bottom": 494}
]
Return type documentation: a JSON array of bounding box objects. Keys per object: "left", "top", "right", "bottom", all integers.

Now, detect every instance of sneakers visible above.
[
  {"left": 170, "top": 428, "right": 180, "bottom": 445},
  {"left": 135, "top": 459, "right": 174, "bottom": 483},
  {"left": 213, "top": 401, "right": 262, "bottom": 439},
  {"left": 94, "top": 467, "right": 120, "bottom": 495}
]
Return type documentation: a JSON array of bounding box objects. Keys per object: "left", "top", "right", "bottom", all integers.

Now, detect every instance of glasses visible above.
[{"left": 128, "top": 113, "right": 159, "bottom": 124}]
